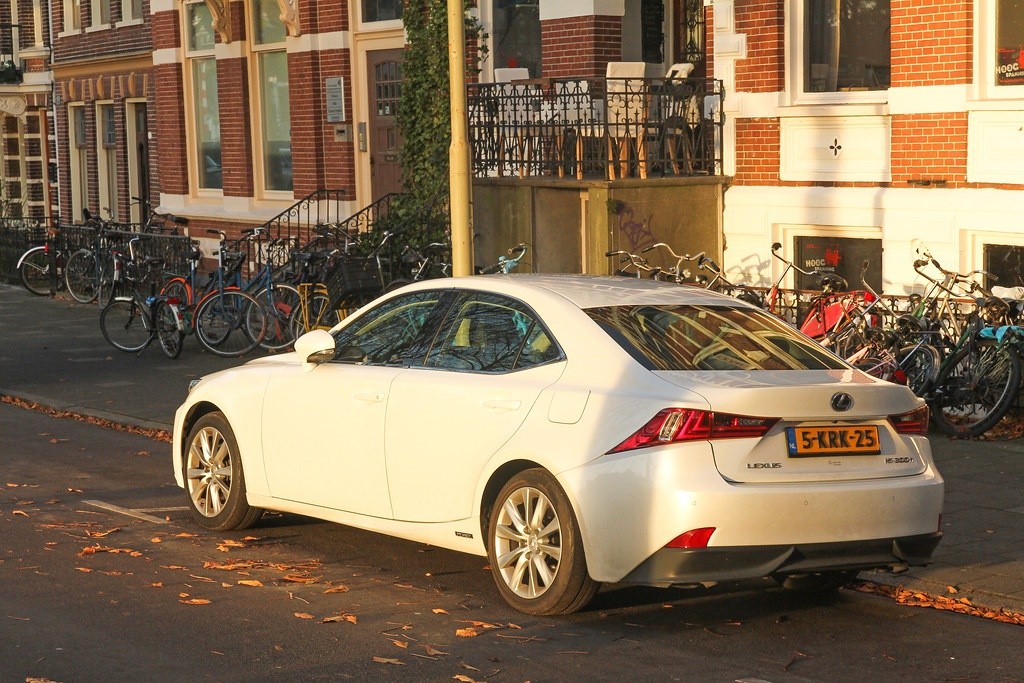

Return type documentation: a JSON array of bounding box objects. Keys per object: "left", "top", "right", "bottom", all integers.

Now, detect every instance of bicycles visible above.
[
  {"left": 18, "top": 194, "right": 526, "bottom": 357},
  {"left": 607, "top": 243, "right": 1023, "bottom": 439}
]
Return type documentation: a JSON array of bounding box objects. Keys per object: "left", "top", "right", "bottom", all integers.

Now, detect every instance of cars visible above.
[{"left": 170, "top": 271, "right": 947, "bottom": 619}]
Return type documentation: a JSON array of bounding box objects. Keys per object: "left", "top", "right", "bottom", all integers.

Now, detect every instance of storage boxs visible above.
[{"left": 324, "top": 257, "right": 389, "bottom": 311}]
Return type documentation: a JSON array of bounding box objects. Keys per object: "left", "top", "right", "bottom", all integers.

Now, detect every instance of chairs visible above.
[
  {"left": 450, "top": 310, "right": 526, "bottom": 371},
  {"left": 495, "top": 61, "right": 694, "bottom": 179}
]
